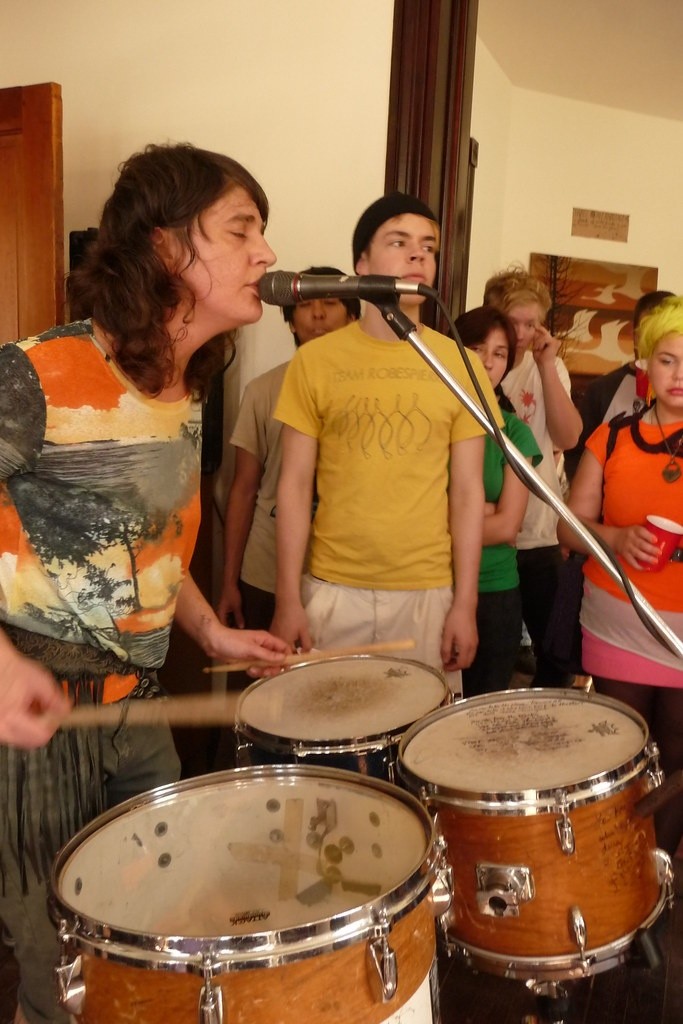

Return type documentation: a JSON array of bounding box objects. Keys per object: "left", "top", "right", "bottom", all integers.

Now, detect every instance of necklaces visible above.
[{"left": 655, "top": 403, "right": 683, "bottom": 483}]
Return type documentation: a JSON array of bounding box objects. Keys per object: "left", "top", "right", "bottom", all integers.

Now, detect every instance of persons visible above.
[
  {"left": 557, "top": 292, "right": 683, "bottom": 856},
  {"left": 269, "top": 193, "right": 506, "bottom": 702},
  {"left": 485, "top": 273, "right": 582, "bottom": 690},
  {"left": 0, "top": 144, "right": 294, "bottom": 1024},
  {"left": 216, "top": 266, "right": 361, "bottom": 768},
  {"left": 451, "top": 305, "right": 543, "bottom": 697}
]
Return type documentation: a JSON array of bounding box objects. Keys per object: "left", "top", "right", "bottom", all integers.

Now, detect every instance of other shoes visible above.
[{"left": 515, "top": 644, "right": 536, "bottom": 676}]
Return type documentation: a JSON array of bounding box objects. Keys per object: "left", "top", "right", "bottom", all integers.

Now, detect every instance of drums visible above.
[
  {"left": 234, "top": 650, "right": 454, "bottom": 803},
  {"left": 389, "top": 686, "right": 664, "bottom": 983},
  {"left": 44, "top": 762, "right": 439, "bottom": 1024}
]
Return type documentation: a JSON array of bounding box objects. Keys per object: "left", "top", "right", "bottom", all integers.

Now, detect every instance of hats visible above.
[{"left": 352, "top": 191, "right": 438, "bottom": 275}]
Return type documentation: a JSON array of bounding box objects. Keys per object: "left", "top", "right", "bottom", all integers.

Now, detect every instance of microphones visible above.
[{"left": 258, "top": 270, "right": 438, "bottom": 307}]
